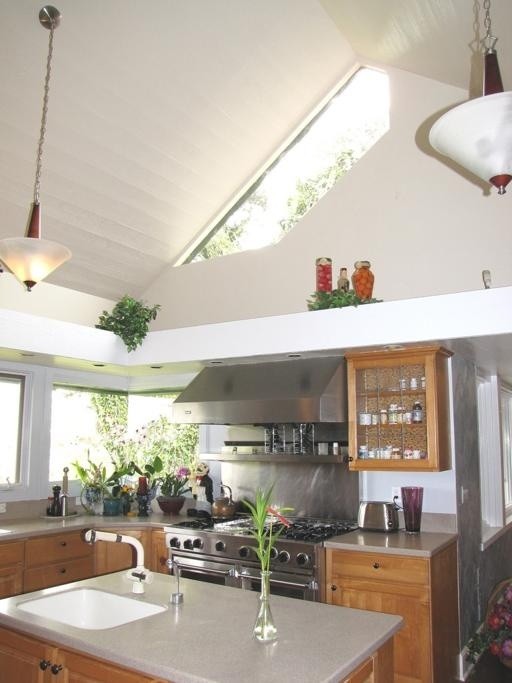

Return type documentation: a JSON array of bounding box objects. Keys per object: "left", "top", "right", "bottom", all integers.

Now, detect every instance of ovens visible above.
[{"left": 169, "top": 555, "right": 321, "bottom": 604}]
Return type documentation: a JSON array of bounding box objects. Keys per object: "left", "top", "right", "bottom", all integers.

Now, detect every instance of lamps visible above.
[
  {"left": 0, "top": 0, "right": 88, "bottom": 295},
  {"left": 420, "top": 0, "right": 512, "bottom": 202}
]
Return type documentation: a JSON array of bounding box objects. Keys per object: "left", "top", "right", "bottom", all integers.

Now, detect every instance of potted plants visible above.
[
  {"left": 67, "top": 445, "right": 192, "bottom": 517},
  {"left": 234, "top": 475, "right": 298, "bottom": 641}
]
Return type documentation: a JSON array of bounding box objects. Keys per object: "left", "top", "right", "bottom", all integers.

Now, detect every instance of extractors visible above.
[{"left": 169, "top": 357, "right": 346, "bottom": 423}]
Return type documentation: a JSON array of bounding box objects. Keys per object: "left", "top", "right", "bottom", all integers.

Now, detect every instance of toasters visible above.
[{"left": 355, "top": 500, "right": 399, "bottom": 533}]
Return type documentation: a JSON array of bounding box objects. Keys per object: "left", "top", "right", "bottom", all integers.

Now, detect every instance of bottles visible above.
[
  {"left": 332, "top": 441, "right": 339, "bottom": 456},
  {"left": 47, "top": 467, "right": 70, "bottom": 515},
  {"left": 338, "top": 266, "right": 349, "bottom": 289},
  {"left": 316, "top": 257, "right": 334, "bottom": 292},
  {"left": 359, "top": 377, "right": 428, "bottom": 459},
  {"left": 352, "top": 259, "right": 374, "bottom": 301},
  {"left": 263, "top": 423, "right": 315, "bottom": 455}
]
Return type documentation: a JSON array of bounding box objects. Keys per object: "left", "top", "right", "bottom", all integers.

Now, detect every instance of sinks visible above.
[{"left": 17, "top": 586, "right": 168, "bottom": 631}]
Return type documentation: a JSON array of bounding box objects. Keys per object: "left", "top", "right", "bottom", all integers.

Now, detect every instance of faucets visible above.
[
  {"left": 84, "top": 529, "right": 153, "bottom": 594},
  {"left": 167, "top": 559, "right": 184, "bottom": 604}
]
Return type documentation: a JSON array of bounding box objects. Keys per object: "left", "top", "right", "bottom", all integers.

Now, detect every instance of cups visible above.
[
  {"left": 402, "top": 487, "right": 424, "bottom": 535},
  {"left": 318, "top": 442, "right": 328, "bottom": 455}
]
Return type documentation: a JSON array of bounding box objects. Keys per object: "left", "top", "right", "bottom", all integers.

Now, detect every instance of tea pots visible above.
[{"left": 208, "top": 485, "right": 235, "bottom": 520}]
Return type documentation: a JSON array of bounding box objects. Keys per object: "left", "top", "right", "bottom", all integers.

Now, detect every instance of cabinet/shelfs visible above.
[
  {"left": 0, "top": 619, "right": 395, "bottom": 683},
  {"left": 24, "top": 527, "right": 93, "bottom": 598},
  {"left": 343, "top": 345, "right": 453, "bottom": 474},
  {"left": 93, "top": 527, "right": 149, "bottom": 574},
  {"left": 148, "top": 530, "right": 166, "bottom": 573},
  {"left": 0, "top": 537, "right": 25, "bottom": 600},
  {"left": 324, "top": 542, "right": 463, "bottom": 683}
]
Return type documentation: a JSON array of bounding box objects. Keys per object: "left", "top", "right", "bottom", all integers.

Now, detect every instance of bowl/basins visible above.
[{"left": 187, "top": 508, "right": 208, "bottom": 517}]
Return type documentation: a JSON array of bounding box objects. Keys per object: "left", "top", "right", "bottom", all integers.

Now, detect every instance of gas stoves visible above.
[{"left": 163, "top": 510, "right": 356, "bottom": 574}]
